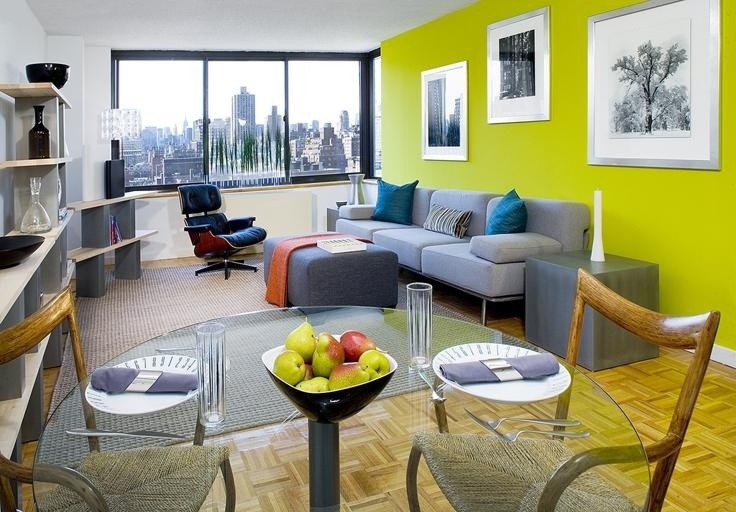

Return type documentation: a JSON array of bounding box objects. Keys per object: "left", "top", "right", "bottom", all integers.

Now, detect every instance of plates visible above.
[
  {"left": 430, "top": 342, "right": 572, "bottom": 405},
  {"left": 84, "top": 353, "right": 212, "bottom": 417}
]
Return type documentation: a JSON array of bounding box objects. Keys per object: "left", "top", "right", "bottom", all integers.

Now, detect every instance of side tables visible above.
[
  {"left": 327, "top": 208, "right": 339, "bottom": 232},
  {"left": 524, "top": 250, "right": 660, "bottom": 373}
]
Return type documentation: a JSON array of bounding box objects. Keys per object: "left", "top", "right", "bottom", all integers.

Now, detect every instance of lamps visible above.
[{"left": 98, "top": 109, "right": 139, "bottom": 160}]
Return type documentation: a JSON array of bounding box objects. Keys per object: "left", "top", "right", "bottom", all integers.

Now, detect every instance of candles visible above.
[{"left": 590, "top": 186, "right": 606, "bottom": 262}]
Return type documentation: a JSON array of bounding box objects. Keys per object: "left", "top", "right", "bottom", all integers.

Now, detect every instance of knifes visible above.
[{"left": 63, "top": 426, "right": 189, "bottom": 442}]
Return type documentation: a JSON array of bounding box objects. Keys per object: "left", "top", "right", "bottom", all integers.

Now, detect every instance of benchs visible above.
[{"left": 263, "top": 231, "right": 399, "bottom": 326}]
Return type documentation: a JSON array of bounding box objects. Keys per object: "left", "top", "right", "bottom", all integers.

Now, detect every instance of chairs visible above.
[
  {"left": 0, "top": 282, "right": 239, "bottom": 512},
  {"left": 407, "top": 268, "right": 721, "bottom": 512},
  {"left": 176, "top": 183, "right": 267, "bottom": 280}
]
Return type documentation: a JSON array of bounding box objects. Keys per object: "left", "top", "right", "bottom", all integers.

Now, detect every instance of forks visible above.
[
  {"left": 461, "top": 408, "right": 581, "bottom": 444},
  {"left": 482, "top": 421, "right": 591, "bottom": 445}
]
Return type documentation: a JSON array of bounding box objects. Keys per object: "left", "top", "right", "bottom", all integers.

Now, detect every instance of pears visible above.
[{"left": 272, "top": 318, "right": 389, "bottom": 394}]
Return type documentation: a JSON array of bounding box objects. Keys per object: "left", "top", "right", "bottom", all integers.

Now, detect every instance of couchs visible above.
[{"left": 335, "top": 188, "right": 591, "bottom": 325}]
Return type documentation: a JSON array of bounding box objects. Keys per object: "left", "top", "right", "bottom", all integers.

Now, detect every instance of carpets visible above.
[{"left": 28, "top": 260, "right": 539, "bottom": 466}]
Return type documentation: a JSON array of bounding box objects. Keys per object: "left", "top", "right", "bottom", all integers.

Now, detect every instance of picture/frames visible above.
[
  {"left": 486, "top": 4, "right": 552, "bottom": 124},
  {"left": 587, "top": 0, "right": 721, "bottom": 172},
  {"left": 420, "top": 60, "right": 469, "bottom": 162}
]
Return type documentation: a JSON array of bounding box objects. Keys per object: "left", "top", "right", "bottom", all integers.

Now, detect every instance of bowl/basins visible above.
[
  {"left": 261, "top": 334, "right": 398, "bottom": 423},
  {"left": 335, "top": 201, "right": 347, "bottom": 207},
  {"left": 25, "top": 62, "right": 70, "bottom": 91},
  {"left": 0, "top": 235, "right": 46, "bottom": 270}
]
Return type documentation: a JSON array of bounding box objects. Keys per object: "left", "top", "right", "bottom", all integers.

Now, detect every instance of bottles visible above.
[{"left": 27, "top": 103, "right": 53, "bottom": 160}]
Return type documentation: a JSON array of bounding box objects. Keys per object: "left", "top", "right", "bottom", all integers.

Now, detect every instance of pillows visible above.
[
  {"left": 468, "top": 232, "right": 563, "bottom": 264},
  {"left": 422, "top": 203, "right": 474, "bottom": 239},
  {"left": 484, "top": 187, "right": 528, "bottom": 236},
  {"left": 371, "top": 177, "right": 419, "bottom": 225},
  {"left": 338, "top": 204, "right": 375, "bottom": 220}
]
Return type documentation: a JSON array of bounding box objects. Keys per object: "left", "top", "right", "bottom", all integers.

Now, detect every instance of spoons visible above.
[{"left": 418, "top": 367, "right": 447, "bottom": 404}]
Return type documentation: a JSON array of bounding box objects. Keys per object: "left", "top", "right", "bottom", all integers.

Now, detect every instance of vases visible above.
[{"left": 28, "top": 104, "right": 50, "bottom": 159}]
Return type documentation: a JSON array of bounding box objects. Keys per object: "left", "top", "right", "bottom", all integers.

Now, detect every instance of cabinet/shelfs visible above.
[
  {"left": 0, "top": 82, "right": 74, "bottom": 462},
  {"left": 67, "top": 185, "right": 162, "bottom": 298}
]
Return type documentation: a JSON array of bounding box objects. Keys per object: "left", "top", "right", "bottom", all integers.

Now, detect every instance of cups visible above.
[
  {"left": 193, "top": 320, "right": 229, "bottom": 427},
  {"left": 404, "top": 281, "right": 434, "bottom": 371}
]
